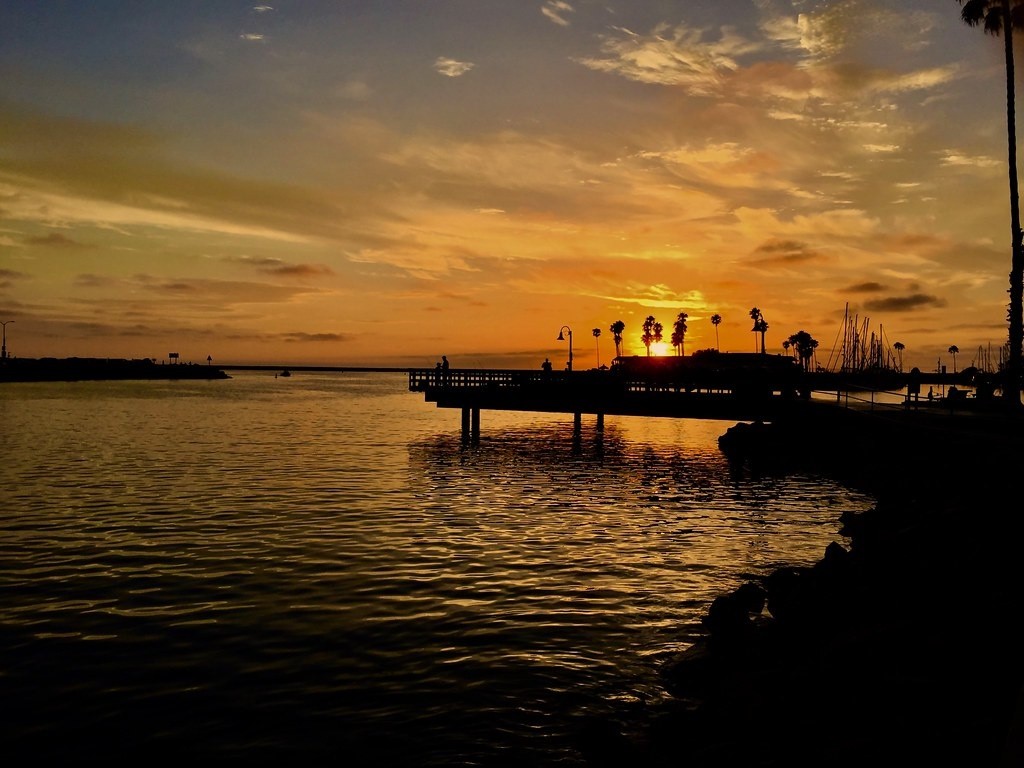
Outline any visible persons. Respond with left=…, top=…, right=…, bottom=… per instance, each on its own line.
left=435, top=355, right=450, bottom=387
left=541, top=357, right=552, bottom=369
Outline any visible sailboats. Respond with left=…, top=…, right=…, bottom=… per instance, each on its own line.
left=799, top=300, right=908, bottom=392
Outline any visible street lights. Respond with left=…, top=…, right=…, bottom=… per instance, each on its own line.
left=557, top=325, right=573, bottom=371
